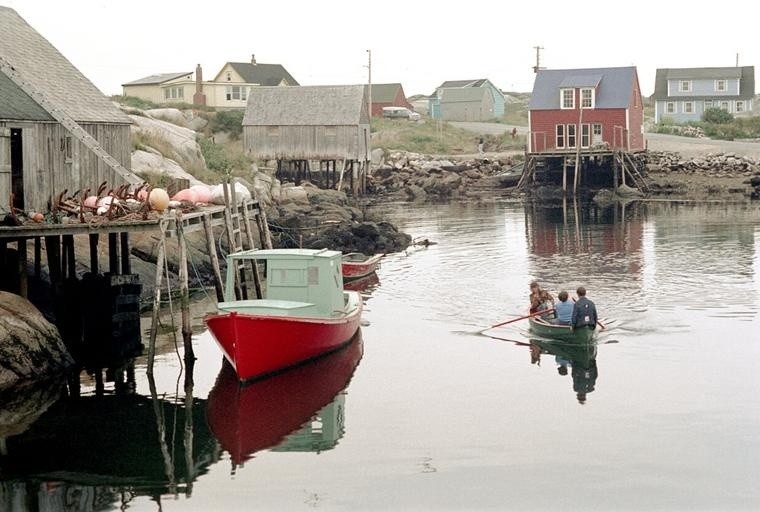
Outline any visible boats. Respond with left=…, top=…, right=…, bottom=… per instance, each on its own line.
left=530, top=309, right=596, bottom=345
left=204, top=250, right=364, bottom=385
left=341, top=253, right=385, bottom=276
left=207, top=329, right=368, bottom=475
left=472, top=296, right=605, bottom=345
left=198, top=245, right=366, bottom=384
left=342, top=253, right=387, bottom=280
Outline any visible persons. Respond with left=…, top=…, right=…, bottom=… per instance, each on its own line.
left=548, top=290, right=574, bottom=326
left=479, top=135, right=484, bottom=155
left=530, top=282, right=553, bottom=314
left=572, top=359, right=597, bottom=400
left=556, top=354, right=572, bottom=375
left=571, top=287, right=597, bottom=330
left=537, top=290, right=555, bottom=319
